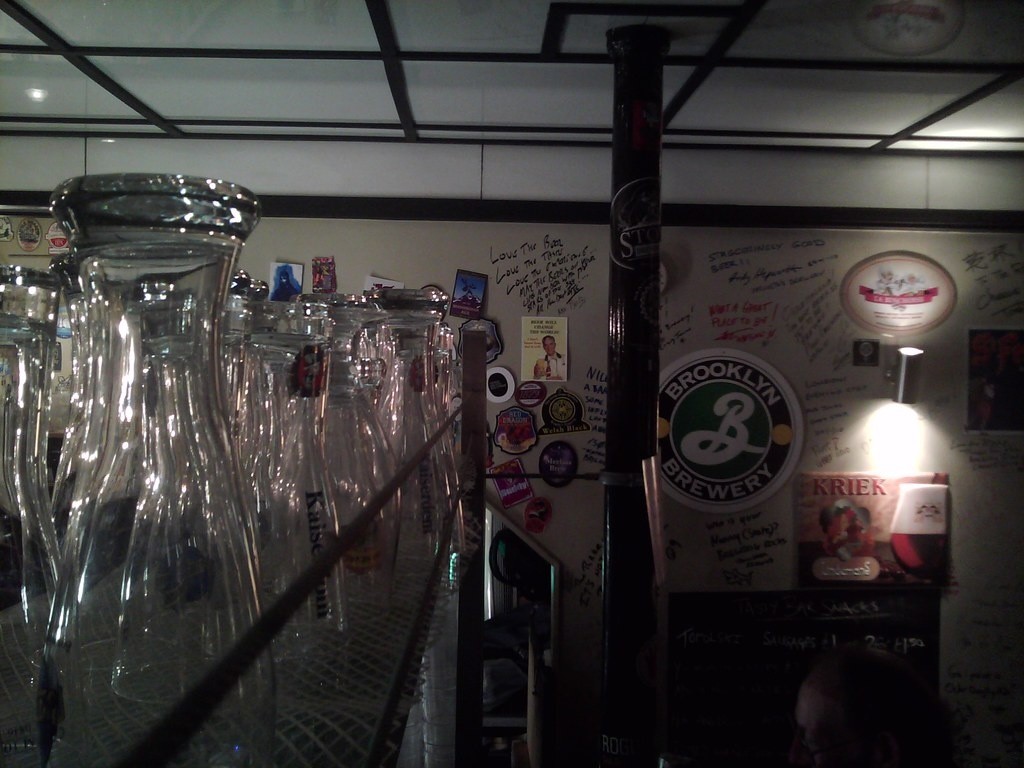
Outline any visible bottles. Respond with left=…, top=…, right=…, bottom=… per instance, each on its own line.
left=0, top=173, right=463, bottom=768
